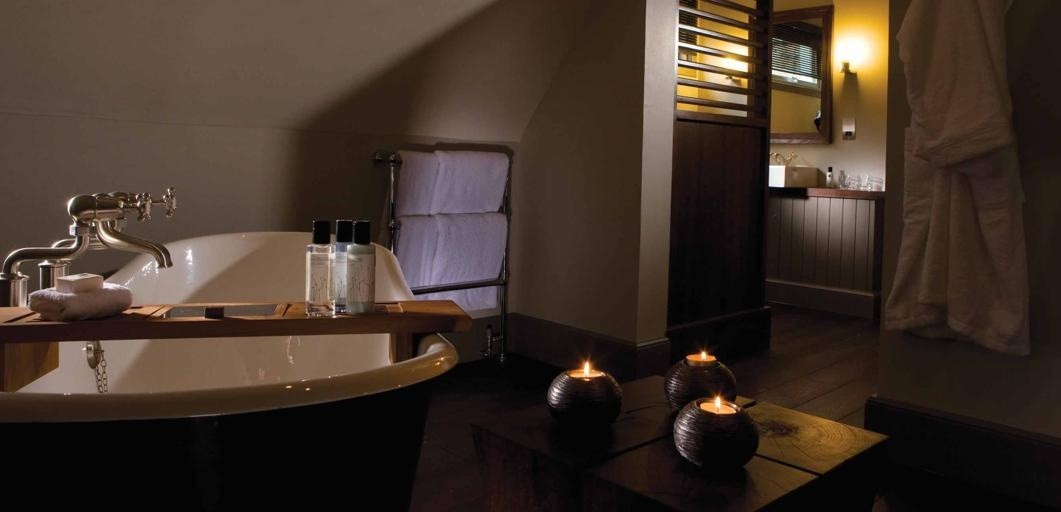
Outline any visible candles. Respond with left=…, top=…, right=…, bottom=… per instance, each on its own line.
left=545, top=353, right=759, bottom=472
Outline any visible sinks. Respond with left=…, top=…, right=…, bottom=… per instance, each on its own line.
left=768, top=164, right=818, bottom=188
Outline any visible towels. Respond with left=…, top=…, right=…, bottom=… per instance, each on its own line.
left=27, top=282, right=133, bottom=322
left=396, top=150, right=510, bottom=310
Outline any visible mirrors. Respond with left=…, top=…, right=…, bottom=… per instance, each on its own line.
left=746, top=3, right=834, bottom=146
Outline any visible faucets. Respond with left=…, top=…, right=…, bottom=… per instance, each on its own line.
left=769, top=152, right=797, bottom=164
left=3, top=190, right=176, bottom=271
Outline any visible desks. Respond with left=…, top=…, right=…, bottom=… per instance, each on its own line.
left=468, top=384, right=894, bottom=510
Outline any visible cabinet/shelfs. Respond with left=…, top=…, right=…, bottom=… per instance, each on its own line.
left=764, top=196, right=886, bottom=321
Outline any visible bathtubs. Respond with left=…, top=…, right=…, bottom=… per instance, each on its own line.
left=0, top=232, right=458, bottom=512
